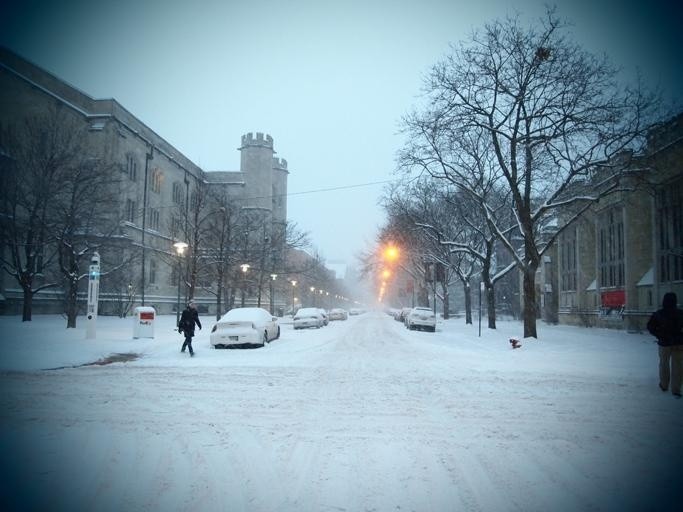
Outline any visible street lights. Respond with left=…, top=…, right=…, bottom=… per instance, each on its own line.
left=237, top=262, right=363, bottom=310
left=169, top=238, right=190, bottom=331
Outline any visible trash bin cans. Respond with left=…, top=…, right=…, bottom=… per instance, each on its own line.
left=133, top=307, right=156, bottom=339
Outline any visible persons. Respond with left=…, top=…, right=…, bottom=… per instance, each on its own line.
left=646, top=291, right=683, bottom=400
left=176, top=301, right=202, bottom=357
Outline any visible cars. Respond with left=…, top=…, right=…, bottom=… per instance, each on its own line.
left=292, top=307, right=366, bottom=331
left=208, top=307, right=282, bottom=349
left=382, top=306, right=437, bottom=332
